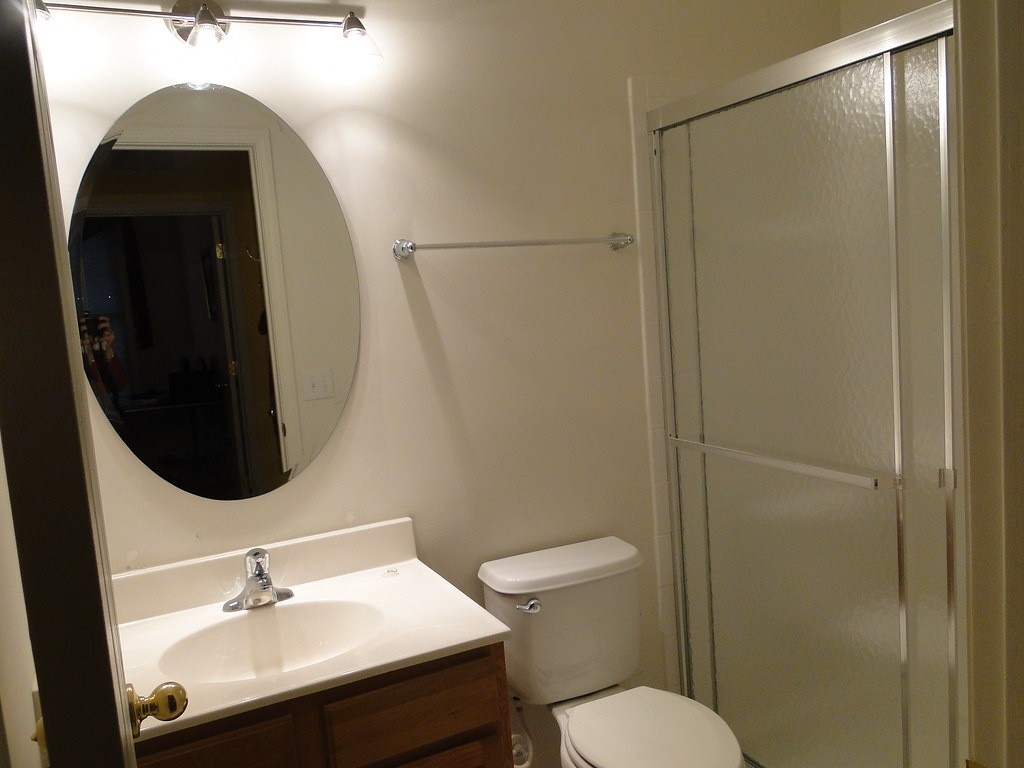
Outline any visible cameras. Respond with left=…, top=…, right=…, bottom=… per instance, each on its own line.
left=85, top=318, right=104, bottom=336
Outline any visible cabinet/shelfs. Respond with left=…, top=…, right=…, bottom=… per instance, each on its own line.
left=133, top=641, right=517, bottom=768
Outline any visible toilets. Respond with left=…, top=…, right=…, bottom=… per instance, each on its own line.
left=477, top=535, right=747, bottom=768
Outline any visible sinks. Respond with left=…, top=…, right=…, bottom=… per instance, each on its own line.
left=158, top=601, right=388, bottom=681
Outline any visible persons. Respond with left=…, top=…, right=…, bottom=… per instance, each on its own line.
left=80, top=315, right=127, bottom=397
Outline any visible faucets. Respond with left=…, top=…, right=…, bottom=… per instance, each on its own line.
left=222, top=548, right=293, bottom=612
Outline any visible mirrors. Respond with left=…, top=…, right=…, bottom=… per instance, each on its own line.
left=68, top=81, right=361, bottom=501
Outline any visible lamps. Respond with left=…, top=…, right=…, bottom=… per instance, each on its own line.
left=35, top=0, right=384, bottom=72
